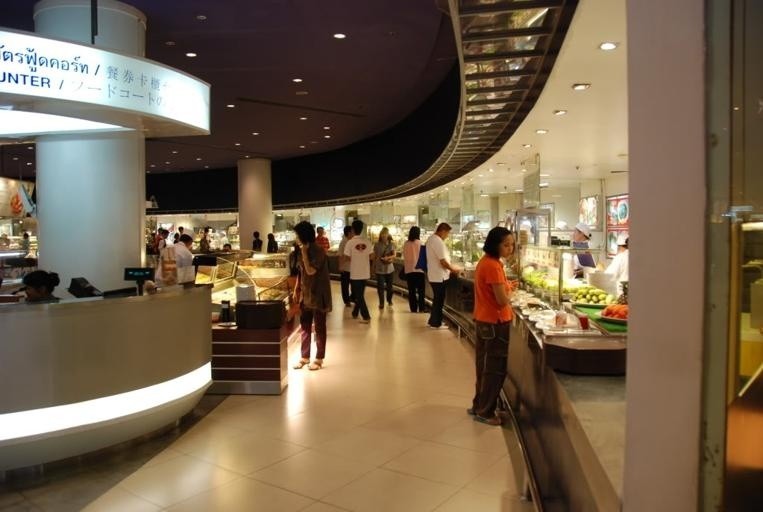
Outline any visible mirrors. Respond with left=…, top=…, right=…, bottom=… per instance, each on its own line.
left=457, top=7, right=554, bottom=150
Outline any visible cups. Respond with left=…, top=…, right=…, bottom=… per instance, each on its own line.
left=551, top=235, right=569, bottom=247
left=211, top=311, right=218, bottom=323
left=520, top=230, right=528, bottom=244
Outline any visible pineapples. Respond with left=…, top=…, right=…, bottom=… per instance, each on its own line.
left=618, top=294, right=628, bottom=304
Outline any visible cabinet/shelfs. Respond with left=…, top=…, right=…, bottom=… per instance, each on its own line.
left=190, top=250, right=304, bottom=397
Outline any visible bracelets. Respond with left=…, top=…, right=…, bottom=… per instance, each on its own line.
left=294, top=287, right=300, bottom=291
left=302, top=258, right=308, bottom=262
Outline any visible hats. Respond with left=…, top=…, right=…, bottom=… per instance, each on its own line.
left=616, top=234, right=628, bottom=246
left=182, top=228, right=196, bottom=240
left=576, top=222, right=592, bottom=239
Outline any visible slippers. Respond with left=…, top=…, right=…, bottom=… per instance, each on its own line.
left=466, top=406, right=504, bottom=425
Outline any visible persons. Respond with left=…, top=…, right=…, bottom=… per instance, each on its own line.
left=604, top=231, right=628, bottom=295
left=0, top=233, right=10, bottom=249
left=22, top=270, right=61, bottom=301
left=18, top=232, right=30, bottom=256
left=468, top=226, right=519, bottom=425
left=292, top=221, right=331, bottom=369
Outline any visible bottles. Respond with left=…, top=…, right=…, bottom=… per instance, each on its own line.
left=232, top=308, right=236, bottom=320
left=220, top=292, right=229, bottom=321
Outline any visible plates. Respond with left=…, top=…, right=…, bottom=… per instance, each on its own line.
left=617, top=200, right=628, bottom=224
left=508, top=280, right=626, bottom=335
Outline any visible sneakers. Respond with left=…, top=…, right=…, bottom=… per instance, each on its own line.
left=426, top=320, right=451, bottom=331
left=360, top=316, right=370, bottom=324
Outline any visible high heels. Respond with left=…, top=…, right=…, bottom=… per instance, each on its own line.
left=292, top=358, right=311, bottom=370
left=307, top=360, right=324, bottom=371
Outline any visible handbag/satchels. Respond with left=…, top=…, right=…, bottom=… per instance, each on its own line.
left=415, top=245, right=427, bottom=271
left=398, top=266, right=407, bottom=281
